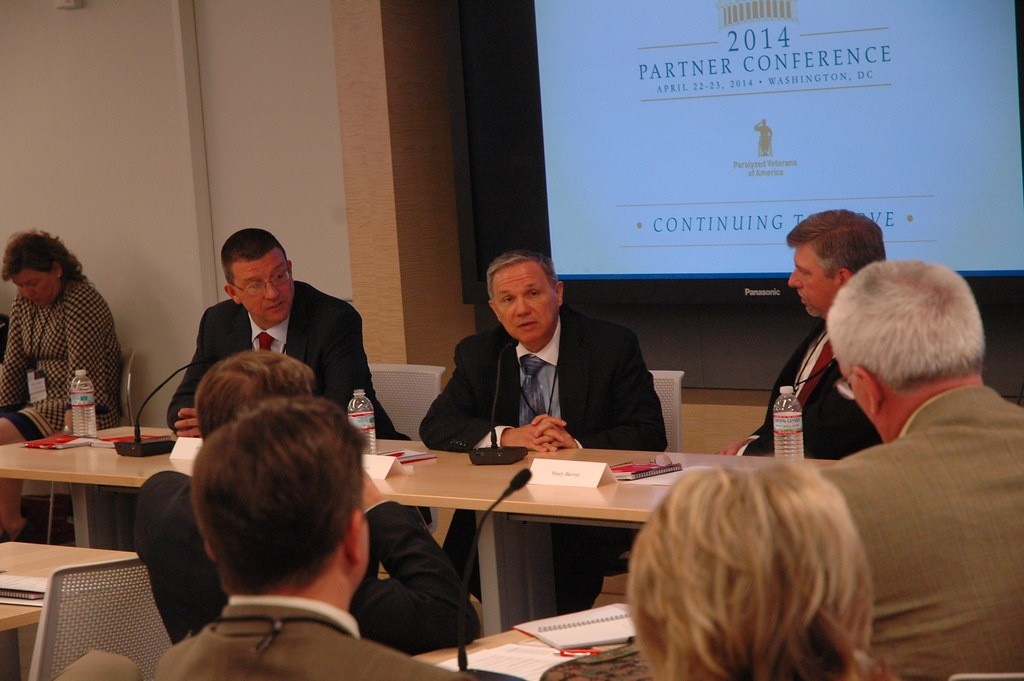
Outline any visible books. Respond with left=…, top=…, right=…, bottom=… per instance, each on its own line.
left=23, top=434, right=99, bottom=450
left=608, top=464, right=682, bottom=480
left=377, top=449, right=438, bottom=465
left=89, top=434, right=172, bottom=448
left=0, top=588, right=44, bottom=601
left=512, top=605, right=637, bottom=652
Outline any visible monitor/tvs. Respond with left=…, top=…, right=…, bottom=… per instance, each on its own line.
left=443, top=0, right=1024, bottom=309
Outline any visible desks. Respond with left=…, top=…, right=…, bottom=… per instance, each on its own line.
left=412, top=630, right=633, bottom=681
left=0, top=540, right=140, bottom=681
left=0, top=427, right=846, bottom=636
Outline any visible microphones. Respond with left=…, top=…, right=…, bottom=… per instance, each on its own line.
left=114, top=355, right=221, bottom=457
left=468, top=339, right=529, bottom=467
left=455, top=467, right=532, bottom=681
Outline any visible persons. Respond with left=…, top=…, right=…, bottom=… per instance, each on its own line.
left=717, top=208, right=887, bottom=459
left=152, top=390, right=478, bottom=681
left=51, top=649, right=145, bottom=681
left=826, top=261, right=1024, bottom=681
left=0, top=230, right=125, bottom=543
left=418, top=249, right=667, bottom=614
left=133, top=350, right=481, bottom=655
left=165, top=227, right=432, bottom=526
left=630, top=461, right=873, bottom=681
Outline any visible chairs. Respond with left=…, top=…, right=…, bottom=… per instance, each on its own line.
left=45, top=343, right=138, bottom=542
left=644, top=369, right=684, bottom=455
left=28, top=555, right=173, bottom=681
left=366, top=363, right=445, bottom=539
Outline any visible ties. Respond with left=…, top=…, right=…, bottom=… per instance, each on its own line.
left=795, top=340, right=834, bottom=404
left=255, top=331, right=275, bottom=352
left=517, top=353, right=548, bottom=428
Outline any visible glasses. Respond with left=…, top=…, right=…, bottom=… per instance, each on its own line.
left=230, top=264, right=292, bottom=295
left=830, top=366, right=860, bottom=399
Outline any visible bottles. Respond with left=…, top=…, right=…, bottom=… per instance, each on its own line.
left=69, top=370, right=98, bottom=438
left=347, top=390, right=377, bottom=455
left=772, top=386, right=804, bottom=462
